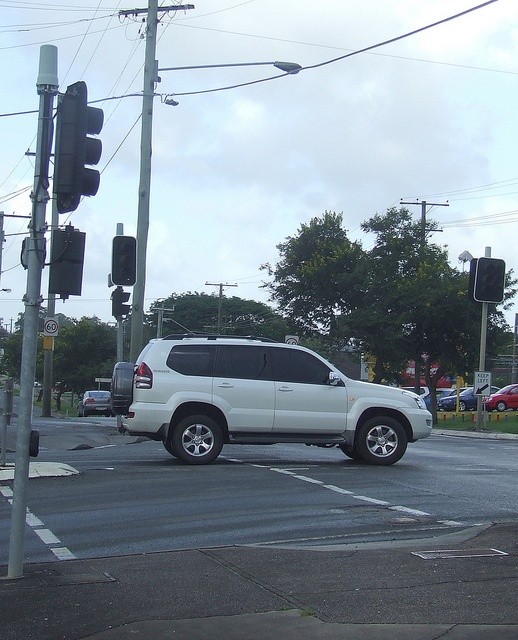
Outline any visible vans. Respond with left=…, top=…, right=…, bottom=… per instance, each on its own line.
left=399, top=387, right=430, bottom=399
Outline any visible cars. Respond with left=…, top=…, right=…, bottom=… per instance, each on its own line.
left=438, top=386, right=500, bottom=411
left=483, top=384, right=518, bottom=412
left=421, top=388, right=453, bottom=411
left=77, top=390, right=112, bottom=417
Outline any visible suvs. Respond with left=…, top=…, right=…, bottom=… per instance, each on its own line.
left=111, top=335, right=432, bottom=464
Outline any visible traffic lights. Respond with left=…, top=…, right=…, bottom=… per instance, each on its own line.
left=111, top=236, right=137, bottom=285
left=468, top=256, right=505, bottom=305
left=49, top=226, right=86, bottom=299
left=53, top=82, right=105, bottom=214
left=111, top=288, right=131, bottom=319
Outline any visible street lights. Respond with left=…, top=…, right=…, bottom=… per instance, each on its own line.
left=128, top=61, right=302, bottom=363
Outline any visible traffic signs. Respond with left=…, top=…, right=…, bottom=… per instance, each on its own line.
left=474, top=371, right=491, bottom=396
left=285, top=336, right=299, bottom=346
left=44, top=318, right=58, bottom=337
